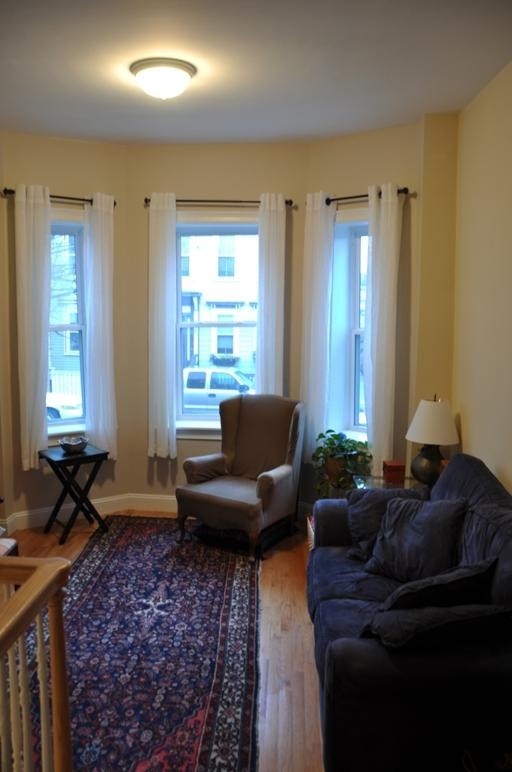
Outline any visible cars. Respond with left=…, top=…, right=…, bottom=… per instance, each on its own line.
left=46, top=391, right=83, bottom=421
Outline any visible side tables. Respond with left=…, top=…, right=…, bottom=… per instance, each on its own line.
left=353, top=474, right=404, bottom=489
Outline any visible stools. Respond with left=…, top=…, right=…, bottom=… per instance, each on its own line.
left=0, top=537, right=21, bottom=591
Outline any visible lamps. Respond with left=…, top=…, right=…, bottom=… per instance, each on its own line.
left=404, top=399, right=461, bottom=485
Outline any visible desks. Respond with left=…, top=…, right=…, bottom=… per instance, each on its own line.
left=39, top=443, right=109, bottom=545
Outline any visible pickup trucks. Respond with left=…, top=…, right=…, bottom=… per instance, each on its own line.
left=181, top=366, right=257, bottom=405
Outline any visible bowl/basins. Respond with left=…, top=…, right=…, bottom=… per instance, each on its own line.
left=57, top=435, right=89, bottom=452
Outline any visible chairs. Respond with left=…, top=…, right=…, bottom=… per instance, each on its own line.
left=175, top=394, right=308, bottom=561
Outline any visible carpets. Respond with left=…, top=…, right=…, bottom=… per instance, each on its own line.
left=0, top=515, right=261, bottom=772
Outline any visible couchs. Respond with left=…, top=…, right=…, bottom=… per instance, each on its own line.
left=307, top=454, right=511, bottom=771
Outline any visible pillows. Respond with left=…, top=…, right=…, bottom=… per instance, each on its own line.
left=372, top=602, right=508, bottom=653
left=360, top=554, right=500, bottom=643
left=365, top=499, right=469, bottom=581
left=345, top=485, right=433, bottom=560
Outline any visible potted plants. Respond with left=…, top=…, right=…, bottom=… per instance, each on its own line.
left=311, top=429, right=373, bottom=498
